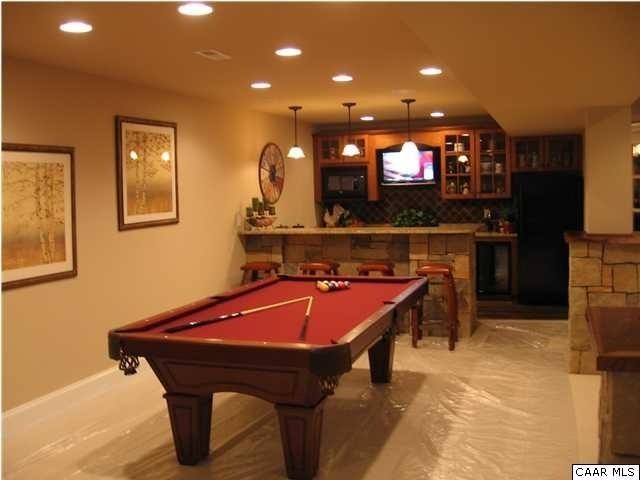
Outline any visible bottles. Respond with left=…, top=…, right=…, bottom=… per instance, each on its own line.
left=479, top=207, right=512, bottom=233
left=518, top=150, right=571, bottom=168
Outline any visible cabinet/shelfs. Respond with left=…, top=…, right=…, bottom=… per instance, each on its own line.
left=311, top=127, right=376, bottom=167
left=440, top=124, right=512, bottom=202
left=510, top=135, right=582, bottom=176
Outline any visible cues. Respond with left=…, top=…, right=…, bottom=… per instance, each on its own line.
left=166, top=295, right=314, bottom=343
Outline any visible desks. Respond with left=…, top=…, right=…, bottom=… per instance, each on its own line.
left=577, top=299, right=640, bottom=464
left=104, top=273, right=435, bottom=480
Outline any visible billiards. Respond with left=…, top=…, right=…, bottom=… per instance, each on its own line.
left=316, top=280, right=351, bottom=292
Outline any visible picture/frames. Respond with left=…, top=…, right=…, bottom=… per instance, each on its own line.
left=1, top=139, right=81, bottom=294
left=110, top=110, right=182, bottom=234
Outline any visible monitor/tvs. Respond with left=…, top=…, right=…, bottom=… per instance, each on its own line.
left=376, top=147, right=442, bottom=188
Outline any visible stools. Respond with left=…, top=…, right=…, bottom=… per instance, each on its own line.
left=353, top=259, right=397, bottom=280
left=292, top=259, right=345, bottom=277
left=408, top=262, right=462, bottom=352
left=239, top=260, right=283, bottom=288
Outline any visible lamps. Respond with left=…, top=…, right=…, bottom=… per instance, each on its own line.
left=338, top=100, right=362, bottom=159
left=399, top=95, right=423, bottom=159
left=286, top=102, right=307, bottom=162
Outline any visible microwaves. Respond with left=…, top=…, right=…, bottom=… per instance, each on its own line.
left=322, top=173, right=365, bottom=197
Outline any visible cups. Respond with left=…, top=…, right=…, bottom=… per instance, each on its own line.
left=445, top=135, right=504, bottom=195
